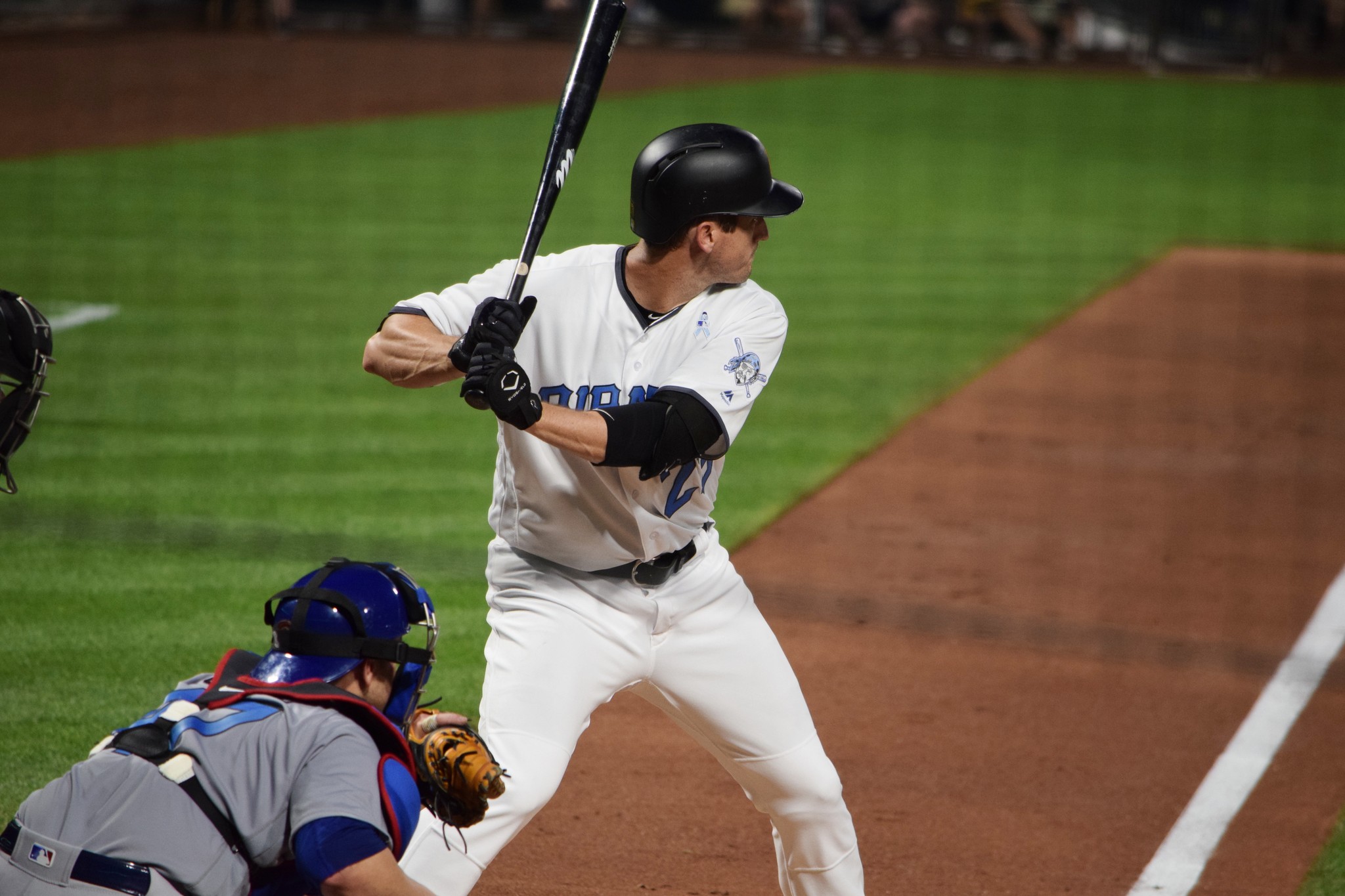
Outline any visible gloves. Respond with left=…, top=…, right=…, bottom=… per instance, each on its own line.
left=460, top=342, right=542, bottom=431
left=447, top=296, right=537, bottom=374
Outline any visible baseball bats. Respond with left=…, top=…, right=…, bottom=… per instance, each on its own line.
left=465, top=1, right=624, bottom=407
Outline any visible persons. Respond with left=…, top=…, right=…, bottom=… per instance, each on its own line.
left=0, top=290, right=52, bottom=494
left=0, top=557, right=507, bottom=896
left=361, top=124, right=865, bottom=896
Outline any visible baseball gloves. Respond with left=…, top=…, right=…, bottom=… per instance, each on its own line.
left=404, top=705, right=509, bottom=828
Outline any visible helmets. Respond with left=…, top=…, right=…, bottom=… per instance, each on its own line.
left=251, top=564, right=411, bottom=684
left=630, top=123, right=804, bottom=244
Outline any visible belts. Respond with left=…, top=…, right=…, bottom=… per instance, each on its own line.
left=0, top=820, right=151, bottom=896
left=586, top=523, right=707, bottom=585
